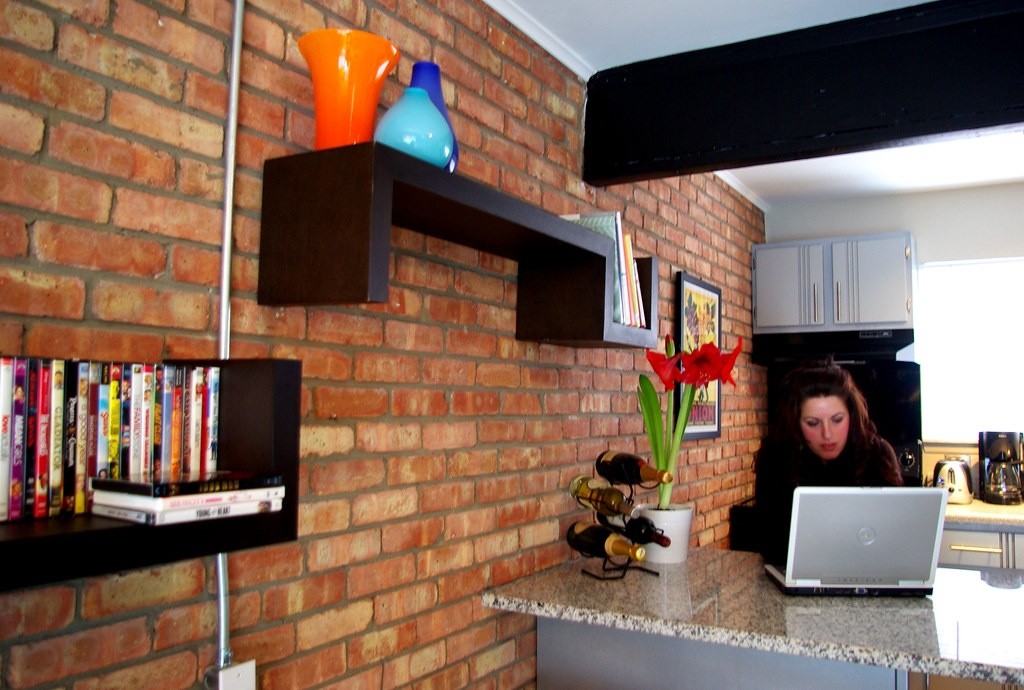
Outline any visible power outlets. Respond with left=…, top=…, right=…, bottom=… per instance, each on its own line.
left=944, top=453, right=971, bottom=466
left=203, top=659, right=257, bottom=690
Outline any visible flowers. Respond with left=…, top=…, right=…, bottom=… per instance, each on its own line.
left=637, top=333, right=744, bottom=508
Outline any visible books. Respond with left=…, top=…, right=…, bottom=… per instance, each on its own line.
left=0, top=355, right=286, bottom=526
left=560, top=212, right=646, bottom=328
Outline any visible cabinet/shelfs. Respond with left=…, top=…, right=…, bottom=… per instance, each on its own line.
left=933, top=493, right=1024, bottom=570
left=749, top=229, right=914, bottom=335
left=253, top=142, right=659, bottom=349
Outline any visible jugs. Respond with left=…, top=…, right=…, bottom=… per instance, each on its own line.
left=985, top=451, right=1022, bottom=496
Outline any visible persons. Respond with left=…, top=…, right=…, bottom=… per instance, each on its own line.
left=751, top=362, right=903, bottom=558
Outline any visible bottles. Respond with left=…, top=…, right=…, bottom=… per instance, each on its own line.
left=596, top=511, right=672, bottom=548
left=595, top=448, right=674, bottom=486
left=565, top=521, right=646, bottom=563
left=567, top=474, right=640, bottom=521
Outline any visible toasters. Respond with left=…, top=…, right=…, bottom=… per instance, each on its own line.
left=933, top=456, right=975, bottom=505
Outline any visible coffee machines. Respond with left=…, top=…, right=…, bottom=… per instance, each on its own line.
left=978, top=432, right=1024, bottom=504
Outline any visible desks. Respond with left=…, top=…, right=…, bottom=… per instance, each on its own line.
left=482, top=547, right=1024, bottom=690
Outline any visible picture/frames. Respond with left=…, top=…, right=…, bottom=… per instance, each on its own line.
left=674, top=270, right=723, bottom=442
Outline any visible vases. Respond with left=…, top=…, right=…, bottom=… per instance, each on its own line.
left=299, top=27, right=401, bottom=148
left=411, top=60, right=460, bottom=173
left=637, top=503, right=694, bottom=564
left=373, top=88, right=454, bottom=168
left=640, top=561, right=694, bottom=624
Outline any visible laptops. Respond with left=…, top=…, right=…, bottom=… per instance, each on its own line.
left=764, top=487, right=949, bottom=596
